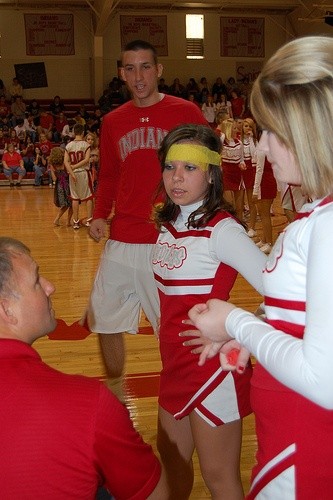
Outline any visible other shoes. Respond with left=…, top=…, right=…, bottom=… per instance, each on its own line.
left=247, top=229, right=257, bottom=238
left=72, top=218, right=80, bottom=229
left=9, top=182, right=21, bottom=187
left=256, top=240, right=273, bottom=255
left=85, top=216, right=94, bottom=227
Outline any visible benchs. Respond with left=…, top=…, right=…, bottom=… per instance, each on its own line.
left=0, top=98, right=123, bottom=186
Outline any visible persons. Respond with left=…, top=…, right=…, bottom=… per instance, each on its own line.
left=187, top=35, right=333, bottom=500
left=49, top=123, right=100, bottom=229
left=147, top=123, right=269, bottom=500
left=0, top=76, right=126, bottom=186
left=0, top=235, right=171, bottom=500
left=158, top=75, right=309, bottom=254
left=87, top=39, right=212, bottom=405
